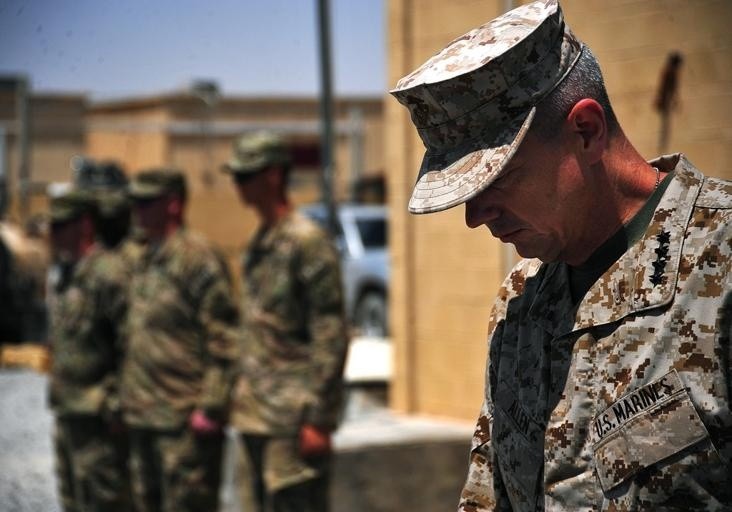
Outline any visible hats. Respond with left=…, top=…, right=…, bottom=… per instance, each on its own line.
left=389, top=0, right=584, bottom=214
left=220, top=132, right=296, bottom=174
left=47, top=162, right=185, bottom=223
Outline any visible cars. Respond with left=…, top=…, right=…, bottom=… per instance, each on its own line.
left=299, top=202, right=391, bottom=338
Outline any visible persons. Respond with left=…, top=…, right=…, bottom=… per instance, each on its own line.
left=113, top=168, right=244, bottom=512
left=391, top=0, right=731, bottom=511
left=218, top=128, right=353, bottom=512
left=34, top=182, right=135, bottom=512
